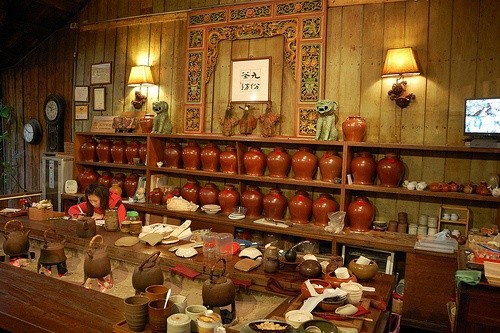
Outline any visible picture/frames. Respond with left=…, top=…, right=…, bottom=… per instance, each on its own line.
left=91, top=116, right=117, bottom=133
left=93, top=87, right=107, bottom=112
left=73, top=85, right=90, bottom=103
left=90, top=61, right=112, bottom=86
left=229, top=56, right=273, bottom=105
left=341, top=244, right=395, bottom=275
left=74, top=104, right=90, bottom=120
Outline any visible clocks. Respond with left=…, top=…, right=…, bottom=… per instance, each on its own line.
left=43, top=94, right=66, bottom=157
left=21, top=119, right=43, bottom=145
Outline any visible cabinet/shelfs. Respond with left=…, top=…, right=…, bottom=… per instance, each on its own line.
left=452, top=250, right=500, bottom=333
left=59, top=130, right=500, bottom=257
left=438, top=204, right=471, bottom=239
left=42, top=155, right=74, bottom=212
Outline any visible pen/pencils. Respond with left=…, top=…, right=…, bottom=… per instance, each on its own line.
left=78, top=206, right=83, bottom=213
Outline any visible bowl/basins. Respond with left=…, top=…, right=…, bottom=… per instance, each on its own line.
left=239, top=256, right=378, bottom=333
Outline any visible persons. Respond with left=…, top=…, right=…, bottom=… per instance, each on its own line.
left=68, top=184, right=126, bottom=222
left=220, top=101, right=278, bottom=137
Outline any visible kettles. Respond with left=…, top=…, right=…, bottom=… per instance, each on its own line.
left=3, top=220, right=31, bottom=256
left=202, top=258, right=236, bottom=308
left=76, top=213, right=96, bottom=238
left=84, top=234, right=111, bottom=278
left=132, top=251, right=164, bottom=292
left=38, top=228, right=67, bottom=265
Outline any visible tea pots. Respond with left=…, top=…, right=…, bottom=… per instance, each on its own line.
left=403, top=179, right=500, bottom=197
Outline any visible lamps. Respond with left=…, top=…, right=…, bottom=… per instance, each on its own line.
left=127, top=64, right=154, bottom=110
left=381, top=47, right=421, bottom=109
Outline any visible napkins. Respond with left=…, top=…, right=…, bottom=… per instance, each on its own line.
left=323, top=286, right=348, bottom=298
left=140, top=225, right=166, bottom=247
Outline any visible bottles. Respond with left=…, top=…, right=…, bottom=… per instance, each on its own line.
left=263, top=246, right=279, bottom=273
left=139, top=114, right=154, bottom=133
left=78, top=138, right=342, bottom=254
left=342, top=116, right=366, bottom=142
left=442, top=213, right=460, bottom=238
left=351, top=151, right=405, bottom=188
left=285, top=248, right=296, bottom=262
left=346, top=195, right=374, bottom=232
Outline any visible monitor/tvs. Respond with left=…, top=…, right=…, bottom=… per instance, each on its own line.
left=463, top=97, right=500, bottom=137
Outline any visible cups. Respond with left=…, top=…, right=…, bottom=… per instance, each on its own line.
left=120, top=221, right=130, bottom=233
left=130, top=221, right=142, bottom=237
left=372, top=213, right=438, bottom=237
left=123, top=285, right=226, bottom=333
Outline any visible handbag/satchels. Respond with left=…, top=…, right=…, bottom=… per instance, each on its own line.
left=148, top=171, right=168, bottom=202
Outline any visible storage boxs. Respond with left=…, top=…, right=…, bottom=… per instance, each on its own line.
left=203, top=232, right=234, bottom=265
left=28, top=207, right=54, bottom=222
left=392, top=298, right=404, bottom=316
